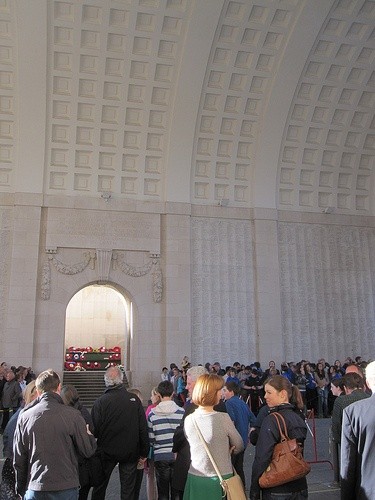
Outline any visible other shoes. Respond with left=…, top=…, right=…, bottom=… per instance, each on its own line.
left=327, top=480, right=339, bottom=489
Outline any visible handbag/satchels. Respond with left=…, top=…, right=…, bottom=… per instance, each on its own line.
left=221, top=474, right=248, bottom=500
left=258, top=412, right=311, bottom=487
